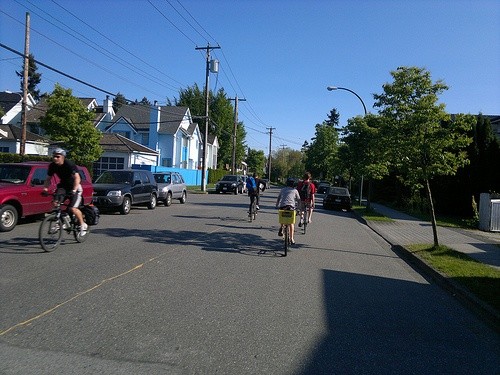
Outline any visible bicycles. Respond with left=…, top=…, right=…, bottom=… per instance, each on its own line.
left=247, top=189, right=263, bottom=221
left=298, top=204, right=310, bottom=234
left=38, top=191, right=90, bottom=252
left=273, top=206, right=300, bottom=256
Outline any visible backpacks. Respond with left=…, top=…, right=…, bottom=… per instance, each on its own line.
left=301, top=185, right=311, bottom=199
left=246, top=177, right=257, bottom=191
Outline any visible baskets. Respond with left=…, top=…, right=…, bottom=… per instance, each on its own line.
left=278, top=209, right=295, bottom=224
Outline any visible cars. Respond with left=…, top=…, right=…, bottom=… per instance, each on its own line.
left=323, top=186, right=352, bottom=209
left=317, top=183, right=330, bottom=193
left=259, top=179, right=270, bottom=190
left=152, top=172, right=188, bottom=207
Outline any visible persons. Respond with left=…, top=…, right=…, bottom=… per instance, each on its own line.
left=248, top=173, right=266, bottom=217
left=297, top=172, right=315, bottom=227
left=276, top=177, right=301, bottom=244
left=43, top=148, right=88, bottom=236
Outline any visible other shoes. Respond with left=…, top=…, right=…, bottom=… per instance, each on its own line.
left=278, top=227, right=283, bottom=236
left=256, top=207, right=260, bottom=210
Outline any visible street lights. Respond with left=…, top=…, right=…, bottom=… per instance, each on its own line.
left=327, top=85, right=368, bottom=206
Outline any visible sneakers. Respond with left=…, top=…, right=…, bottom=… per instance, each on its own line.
left=50, top=223, right=66, bottom=229
left=79, top=222, right=88, bottom=237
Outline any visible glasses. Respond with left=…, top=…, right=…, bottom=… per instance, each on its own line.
left=53, top=155, right=60, bottom=158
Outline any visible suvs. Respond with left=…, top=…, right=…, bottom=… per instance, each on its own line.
left=241, top=175, right=248, bottom=193
left=216, top=174, right=244, bottom=195
left=92, top=168, right=158, bottom=215
left=0, top=160, right=94, bottom=232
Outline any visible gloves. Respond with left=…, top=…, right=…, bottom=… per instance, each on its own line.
left=40, top=191, right=48, bottom=196
left=69, top=190, right=76, bottom=196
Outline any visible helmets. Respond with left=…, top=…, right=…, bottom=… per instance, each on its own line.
left=53, top=148, right=66, bottom=156
